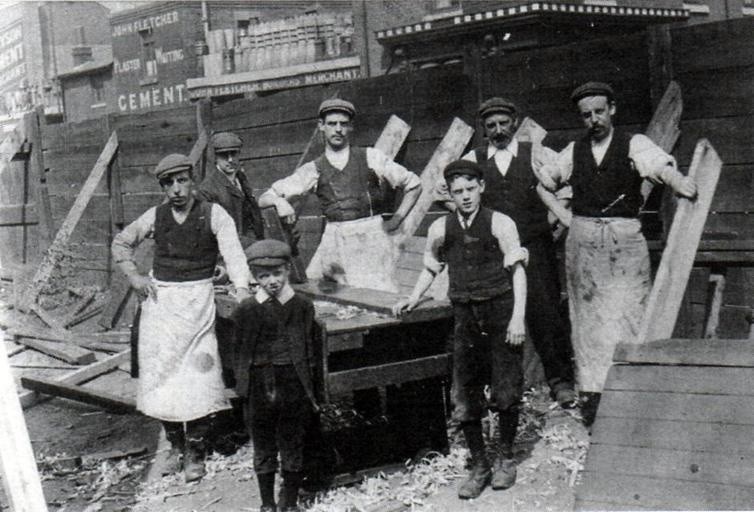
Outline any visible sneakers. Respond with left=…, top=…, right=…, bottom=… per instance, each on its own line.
left=444, top=161, right=483, bottom=177
left=478, top=97, right=517, bottom=115
left=317, top=99, right=355, bottom=116
left=577, top=392, right=601, bottom=425
left=571, top=82, right=614, bottom=101
left=246, top=239, right=291, bottom=266
left=555, top=388, right=575, bottom=406
left=212, top=132, right=242, bottom=153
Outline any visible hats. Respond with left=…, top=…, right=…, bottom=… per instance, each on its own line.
left=156, top=154, right=191, bottom=178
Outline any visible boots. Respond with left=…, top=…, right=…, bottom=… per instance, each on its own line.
left=258, top=472, right=277, bottom=511
left=185, top=427, right=206, bottom=482
left=162, top=430, right=187, bottom=475
left=279, top=470, right=300, bottom=511
left=490, top=412, right=518, bottom=490
left=458, top=421, right=491, bottom=499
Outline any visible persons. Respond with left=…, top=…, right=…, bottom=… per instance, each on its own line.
left=390, top=158, right=530, bottom=500
left=535, top=80, right=698, bottom=435
left=430, top=93, right=574, bottom=407
left=108, top=152, right=255, bottom=482
left=192, top=131, right=264, bottom=280
left=229, top=239, right=318, bottom=511
left=258, top=96, right=421, bottom=290
